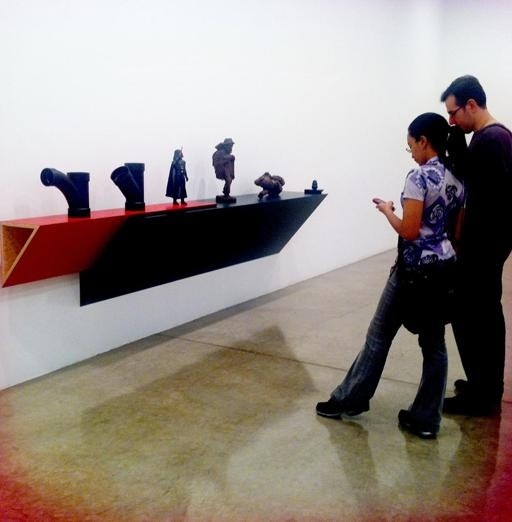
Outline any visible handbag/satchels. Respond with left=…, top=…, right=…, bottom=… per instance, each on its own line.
left=390, top=263, right=453, bottom=335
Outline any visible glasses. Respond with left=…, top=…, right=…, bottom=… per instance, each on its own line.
left=447, top=105, right=467, bottom=117
left=405, top=137, right=417, bottom=154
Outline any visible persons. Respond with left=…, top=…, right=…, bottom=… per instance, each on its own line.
left=214, top=136, right=236, bottom=198
left=166, top=147, right=189, bottom=206
left=438, top=72, right=511, bottom=415
left=316, top=110, right=466, bottom=440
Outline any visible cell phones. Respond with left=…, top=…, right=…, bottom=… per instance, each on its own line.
left=372, top=197, right=395, bottom=211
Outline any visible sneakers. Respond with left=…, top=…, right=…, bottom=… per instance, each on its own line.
left=442, top=379, right=503, bottom=417
left=399, top=406, right=442, bottom=436
left=315, top=394, right=371, bottom=419
